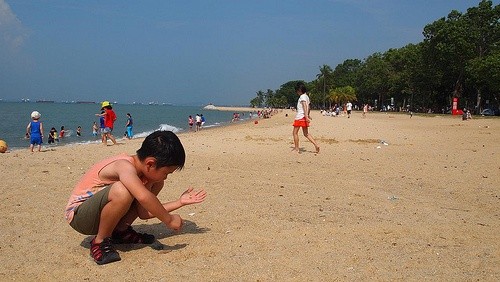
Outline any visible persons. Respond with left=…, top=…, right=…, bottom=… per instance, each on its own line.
left=76, top=126, right=81, bottom=137
left=128, top=114, right=133, bottom=139
left=65, top=130, right=208, bottom=264
left=27, top=110, right=44, bottom=154
left=318, top=95, right=500, bottom=122
left=92, top=121, right=100, bottom=137
left=58, top=125, right=69, bottom=141
left=125, top=113, right=134, bottom=140
left=99, top=111, right=110, bottom=146
left=25, top=132, right=31, bottom=139
left=47, top=127, right=55, bottom=145
left=186, top=112, right=207, bottom=131
left=230, top=102, right=298, bottom=123
left=291, top=81, right=320, bottom=154
left=123, top=130, right=129, bottom=139
left=49, top=128, right=59, bottom=146
left=94, top=100, right=118, bottom=146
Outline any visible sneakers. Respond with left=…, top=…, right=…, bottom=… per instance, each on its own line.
left=88, top=234, right=120, bottom=265
left=112, top=228, right=157, bottom=248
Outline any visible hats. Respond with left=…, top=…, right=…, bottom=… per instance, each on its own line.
left=100, top=101, right=112, bottom=110
left=31, top=111, right=43, bottom=120
